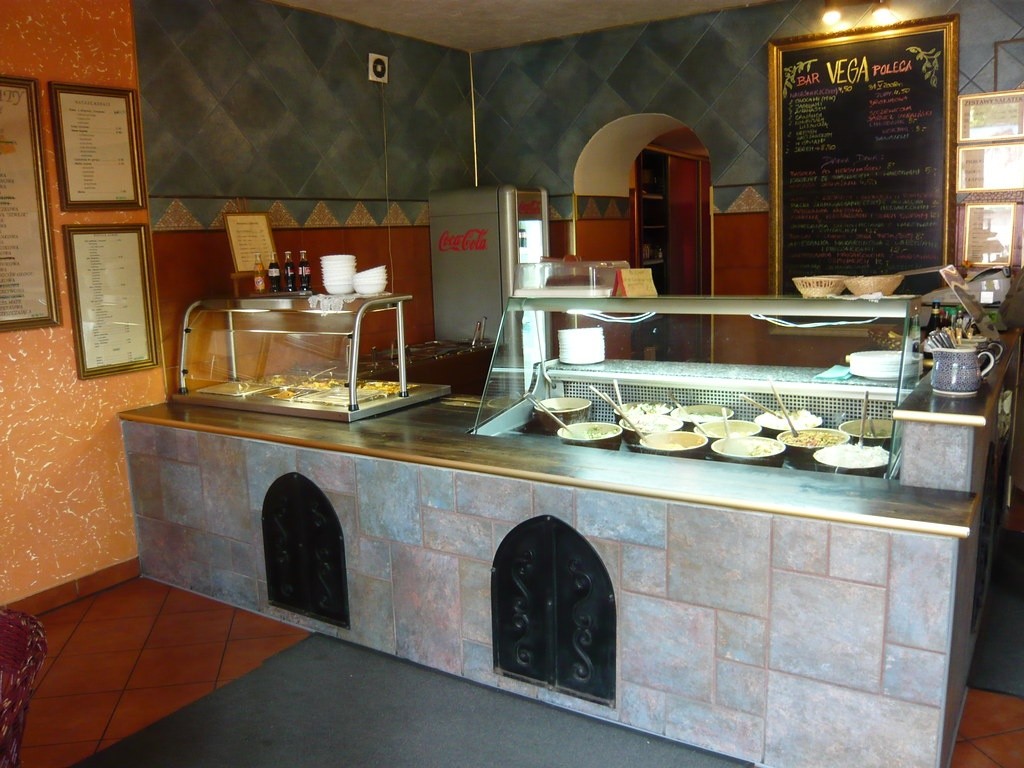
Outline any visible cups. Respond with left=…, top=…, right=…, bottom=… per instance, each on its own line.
left=929, top=335, right=1002, bottom=394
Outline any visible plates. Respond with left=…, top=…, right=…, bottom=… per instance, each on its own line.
left=849, top=351, right=923, bottom=382
left=557, top=328, right=605, bottom=365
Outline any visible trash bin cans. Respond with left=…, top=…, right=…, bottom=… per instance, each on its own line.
left=0, top=605, right=46, bottom=768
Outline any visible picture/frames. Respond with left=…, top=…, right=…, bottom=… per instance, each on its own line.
left=955, top=142, right=1024, bottom=193
left=963, top=201, right=1015, bottom=267
left=46, top=81, right=147, bottom=208
left=62, top=222, right=160, bottom=380
left=956, top=88, right=1024, bottom=143
left=0, top=73, right=62, bottom=333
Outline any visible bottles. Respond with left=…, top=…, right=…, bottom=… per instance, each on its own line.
left=254, top=252, right=266, bottom=295
left=923, top=300, right=964, bottom=359
left=298, top=250, right=311, bottom=290
left=283, top=251, right=296, bottom=291
left=268, top=252, right=282, bottom=293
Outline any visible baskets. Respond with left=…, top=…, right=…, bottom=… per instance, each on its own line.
left=843, top=274, right=904, bottom=296
left=792, top=274, right=850, bottom=298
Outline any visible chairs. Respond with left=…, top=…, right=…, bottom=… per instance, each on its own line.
left=0, top=608, right=48, bottom=768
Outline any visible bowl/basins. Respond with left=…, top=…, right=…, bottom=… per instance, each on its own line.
left=320, top=255, right=388, bottom=294
left=533, top=396, right=894, bottom=478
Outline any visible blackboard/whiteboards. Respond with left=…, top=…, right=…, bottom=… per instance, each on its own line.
left=769, top=12, right=960, bottom=296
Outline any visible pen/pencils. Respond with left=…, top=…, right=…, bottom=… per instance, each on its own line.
left=927, top=327, right=957, bottom=349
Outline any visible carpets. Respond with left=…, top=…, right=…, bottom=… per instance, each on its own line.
left=60, top=633, right=754, bottom=768
left=967, top=527, right=1023, bottom=699
